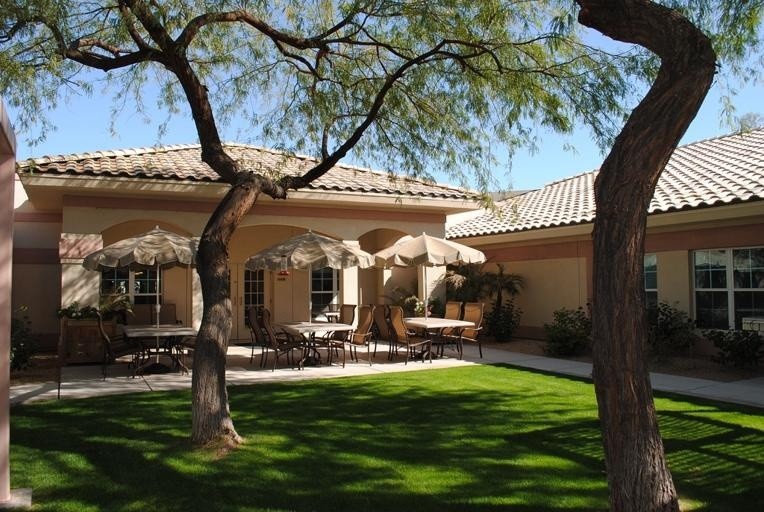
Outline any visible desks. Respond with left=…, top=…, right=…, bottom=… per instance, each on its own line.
left=275, top=321, right=354, bottom=370
left=119, top=319, right=196, bottom=378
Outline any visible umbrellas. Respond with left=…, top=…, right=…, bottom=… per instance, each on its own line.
left=83, top=223, right=198, bottom=362
left=241, top=228, right=374, bottom=354
left=370, top=230, right=487, bottom=348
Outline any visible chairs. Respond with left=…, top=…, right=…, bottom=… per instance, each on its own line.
left=319, top=300, right=486, bottom=369
left=92, top=307, right=144, bottom=381
left=247, top=305, right=301, bottom=370
